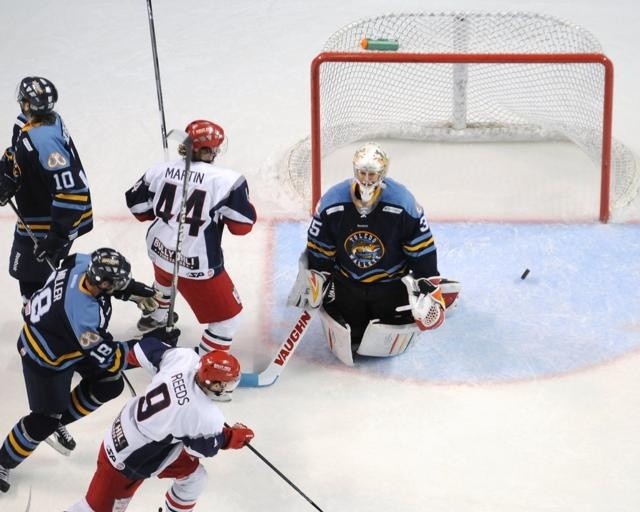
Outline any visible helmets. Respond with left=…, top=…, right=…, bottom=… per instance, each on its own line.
left=354, top=142, right=389, bottom=205
left=87, top=249, right=130, bottom=288
left=196, top=350, right=242, bottom=395
left=17, top=77, right=57, bottom=116
left=186, top=120, right=227, bottom=157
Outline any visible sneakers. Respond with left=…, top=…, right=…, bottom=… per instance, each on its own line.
left=55, top=423, right=76, bottom=450
left=0, top=464, right=10, bottom=493
left=138, top=312, right=179, bottom=331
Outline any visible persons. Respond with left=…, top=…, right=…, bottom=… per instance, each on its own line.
left=125, top=118, right=257, bottom=360
left=0, top=76, right=95, bottom=307
left=0, top=245, right=163, bottom=493
left=286, top=141, right=460, bottom=365
left=62, top=336, right=255, bottom=512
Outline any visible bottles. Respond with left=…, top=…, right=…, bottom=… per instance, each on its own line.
left=360, top=38, right=399, bottom=51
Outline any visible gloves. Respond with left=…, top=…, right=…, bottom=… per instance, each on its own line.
left=222, top=422, right=253, bottom=448
left=34, top=232, right=73, bottom=263
left=113, top=279, right=163, bottom=310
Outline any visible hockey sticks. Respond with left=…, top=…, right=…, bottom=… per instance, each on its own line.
left=166, top=126, right=194, bottom=333
left=238, top=305, right=318, bottom=388
